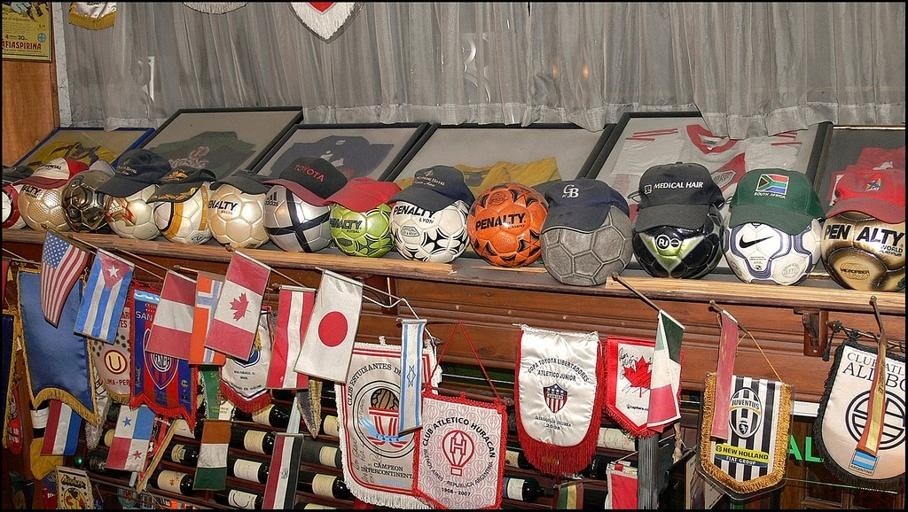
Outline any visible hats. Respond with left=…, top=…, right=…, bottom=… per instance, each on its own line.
left=826, top=163, right=906, bottom=224
left=260, top=155, right=348, bottom=207
left=540, top=178, right=630, bottom=235
left=389, top=164, right=476, bottom=212
left=12, top=156, right=89, bottom=191
left=145, top=165, right=217, bottom=205
left=634, top=160, right=725, bottom=233
left=94, top=148, right=172, bottom=199
left=321, top=176, right=402, bottom=213
left=728, top=167, right=826, bottom=236
left=209, top=169, right=275, bottom=195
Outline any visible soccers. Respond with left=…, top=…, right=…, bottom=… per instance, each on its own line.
left=389, top=200, right=470, bottom=264
left=103, top=183, right=160, bottom=240
left=540, top=204, right=632, bottom=286
left=721, top=211, right=822, bottom=287
left=2, top=182, right=26, bottom=229
left=330, top=203, right=394, bottom=257
left=62, top=178, right=112, bottom=234
left=17, top=182, right=71, bottom=233
left=466, top=182, right=549, bottom=269
left=153, top=182, right=212, bottom=246
left=207, top=185, right=270, bottom=249
left=263, top=184, right=333, bottom=252
left=822, top=210, right=908, bottom=294
left=632, top=203, right=724, bottom=278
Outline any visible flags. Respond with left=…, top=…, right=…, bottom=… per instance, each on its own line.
left=692, top=366, right=792, bottom=506
left=54, top=462, right=96, bottom=509
left=603, top=460, right=639, bottom=509
left=602, top=331, right=680, bottom=443
left=294, top=268, right=365, bottom=386
left=104, top=403, right=156, bottom=473
left=645, top=310, right=686, bottom=427
left=334, top=338, right=439, bottom=509
left=410, top=392, right=509, bottom=510
left=29, top=468, right=59, bottom=509
left=261, top=433, right=305, bottom=509
left=507, top=322, right=606, bottom=478
left=857, top=326, right=888, bottom=457
left=709, top=311, right=738, bottom=441
left=39, top=399, right=82, bottom=458
left=811, top=336, right=906, bottom=489
left=555, top=479, right=584, bottom=510
left=193, top=419, right=233, bottom=492
left=396, top=319, right=427, bottom=436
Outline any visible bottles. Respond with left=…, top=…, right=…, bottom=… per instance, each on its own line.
left=301, top=412, right=341, bottom=437
left=85, top=403, right=159, bottom=482
left=502, top=473, right=558, bottom=503
left=579, top=457, right=637, bottom=480
left=301, top=440, right=342, bottom=469
left=597, top=426, right=637, bottom=452
left=211, top=390, right=297, bottom=509
left=297, top=472, right=355, bottom=499
left=504, top=441, right=534, bottom=469
left=150, top=403, right=206, bottom=497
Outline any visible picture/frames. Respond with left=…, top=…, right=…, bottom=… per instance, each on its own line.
left=385, top=124, right=615, bottom=183
left=251, top=125, right=428, bottom=185
left=138, top=108, right=305, bottom=174
left=587, top=112, right=828, bottom=193
left=812, top=121, right=907, bottom=222
left=12, top=123, right=154, bottom=179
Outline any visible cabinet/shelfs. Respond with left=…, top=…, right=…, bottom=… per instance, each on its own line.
left=64, top=377, right=732, bottom=511
left=0, top=230, right=908, bottom=512
